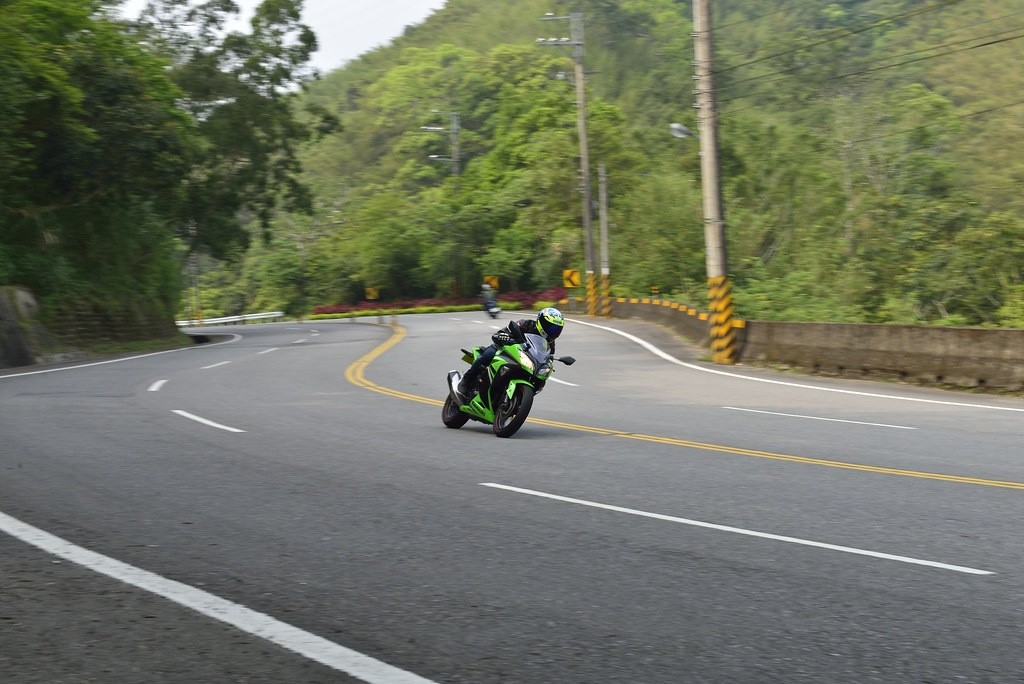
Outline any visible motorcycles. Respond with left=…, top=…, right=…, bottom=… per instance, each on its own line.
left=442, top=320, right=576, bottom=438
left=481, top=295, right=503, bottom=320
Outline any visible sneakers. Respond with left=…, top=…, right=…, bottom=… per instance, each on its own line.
left=456, top=375, right=475, bottom=398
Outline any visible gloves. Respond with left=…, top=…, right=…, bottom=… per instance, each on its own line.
left=498, top=332, right=510, bottom=345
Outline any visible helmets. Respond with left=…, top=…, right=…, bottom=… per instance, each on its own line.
left=535, top=307, right=565, bottom=345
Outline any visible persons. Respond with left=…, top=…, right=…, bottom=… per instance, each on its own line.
left=477, top=283, right=498, bottom=309
left=458, top=307, right=564, bottom=402
